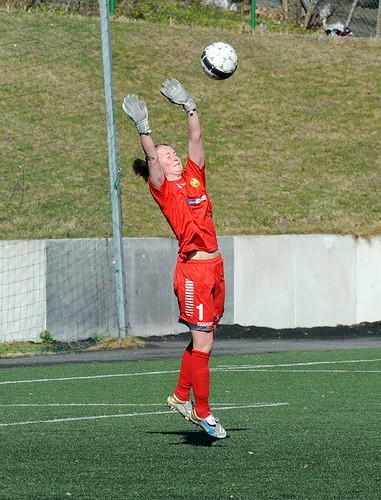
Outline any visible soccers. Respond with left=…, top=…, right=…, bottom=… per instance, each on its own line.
left=199, top=41, right=239, bottom=81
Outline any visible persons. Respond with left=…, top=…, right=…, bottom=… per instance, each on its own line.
left=122, top=77, right=226, bottom=438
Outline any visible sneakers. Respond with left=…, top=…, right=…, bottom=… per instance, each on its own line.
left=166, top=393, right=194, bottom=421
left=190, top=408, right=226, bottom=437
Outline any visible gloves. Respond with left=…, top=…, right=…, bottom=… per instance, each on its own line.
left=122, top=93, right=152, bottom=135
left=159, top=78, right=197, bottom=114
